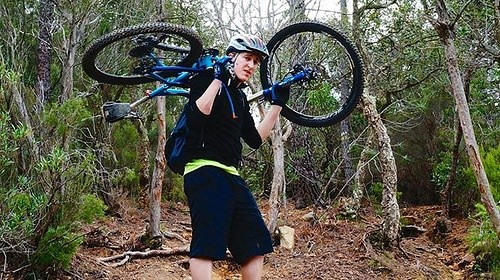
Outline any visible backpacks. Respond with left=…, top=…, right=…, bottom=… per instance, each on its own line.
left=165, top=104, right=194, bottom=175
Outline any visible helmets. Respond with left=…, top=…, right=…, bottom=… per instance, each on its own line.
left=226, top=33, right=270, bottom=63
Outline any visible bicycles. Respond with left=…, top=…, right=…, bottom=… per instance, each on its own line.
left=82, top=21, right=364, bottom=128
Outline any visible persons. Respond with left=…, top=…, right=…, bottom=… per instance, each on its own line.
left=183, top=34, right=290, bottom=280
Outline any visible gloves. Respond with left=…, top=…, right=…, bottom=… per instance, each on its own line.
left=273, top=80, right=290, bottom=108
left=213, top=56, right=235, bottom=84
